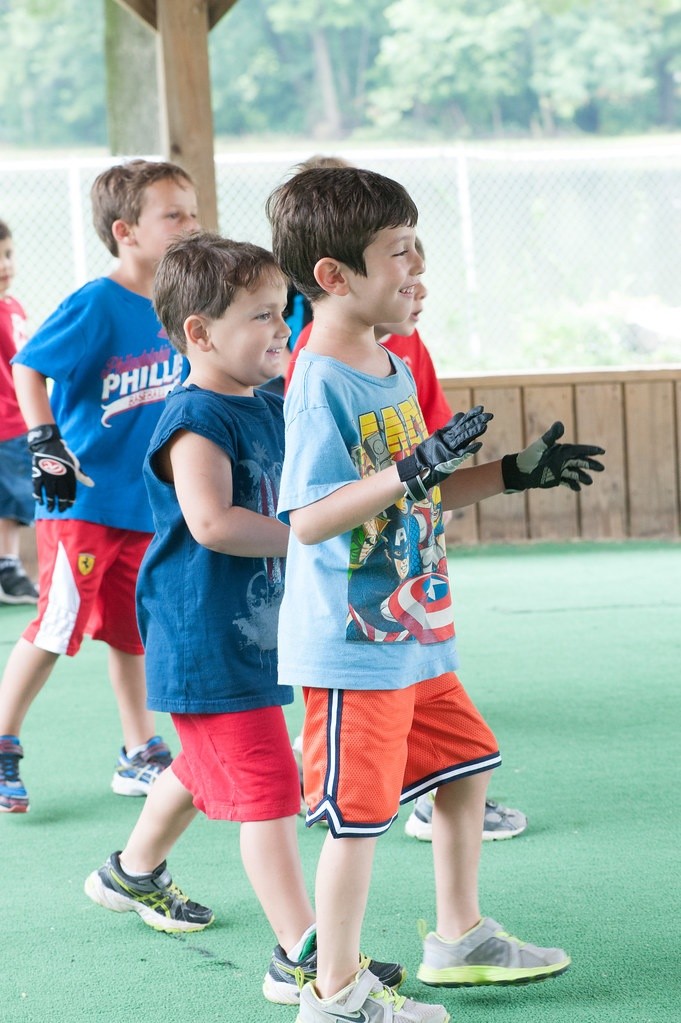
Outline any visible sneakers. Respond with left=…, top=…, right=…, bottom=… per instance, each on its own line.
left=417, top=915, right=570, bottom=988
left=262, top=927, right=406, bottom=1006
left=111, top=736, right=176, bottom=796
left=0, top=734, right=31, bottom=813
left=404, top=789, right=528, bottom=842
left=291, top=749, right=329, bottom=827
left=0, top=559, right=40, bottom=605
left=85, top=850, right=215, bottom=932
left=294, top=967, right=451, bottom=1023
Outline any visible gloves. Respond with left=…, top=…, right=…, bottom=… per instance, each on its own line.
left=27, top=423, right=95, bottom=512
left=396, top=404, right=494, bottom=500
left=501, top=420, right=605, bottom=495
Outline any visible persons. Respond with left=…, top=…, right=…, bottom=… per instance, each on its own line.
left=275, top=155, right=528, bottom=843
left=0, top=222, right=45, bottom=604
left=0, top=160, right=199, bottom=814
left=266, top=167, right=604, bottom=1023
left=83, top=231, right=402, bottom=1006
